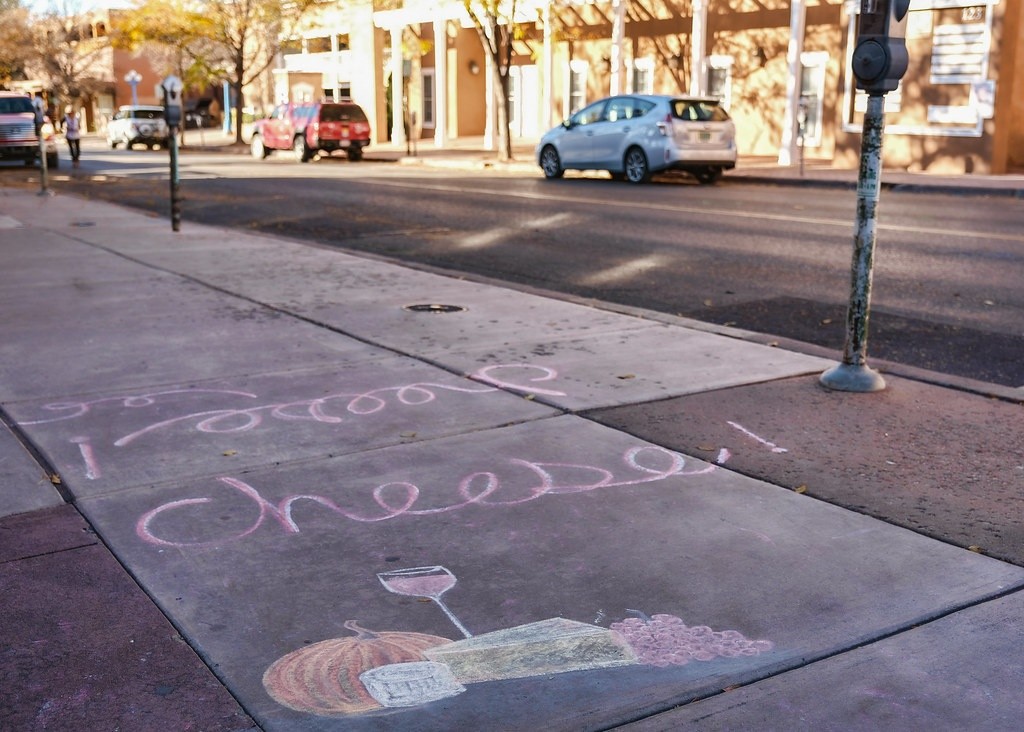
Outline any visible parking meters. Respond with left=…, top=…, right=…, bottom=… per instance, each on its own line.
left=820, top=1, right=912, bottom=392
left=31, top=97, right=54, bottom=197
left=160, top=73, right=184, bottom=233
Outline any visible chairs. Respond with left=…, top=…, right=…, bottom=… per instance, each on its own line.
left=617, top=109, right=627, bottom=121
left=631, top=109, right=642, bottom=118
left=680, top=109, right=690, bottom=119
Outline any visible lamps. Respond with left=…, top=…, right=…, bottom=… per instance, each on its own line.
left=753, top=47, right=762, bottom=66
left=602, top=57, right=610, bottom=72
left=671, top=54, right=680, bottom=68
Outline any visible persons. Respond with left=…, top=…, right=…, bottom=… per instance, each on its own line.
left=58, top=110, right=84, bottom=164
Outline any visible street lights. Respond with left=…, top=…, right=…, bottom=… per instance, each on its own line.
left=212, top=60, right=236, bottom=137
left=123, top=69, right=142, bottom=106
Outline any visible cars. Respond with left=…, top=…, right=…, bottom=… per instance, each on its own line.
left=534, top=92, right=737, bottom=184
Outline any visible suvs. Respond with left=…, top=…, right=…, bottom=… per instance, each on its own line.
left=109, top=105, right=172, bottom=153
left=0, top=90, right=58, bottom=172
left=251, top=101, right=373, bottom=163
